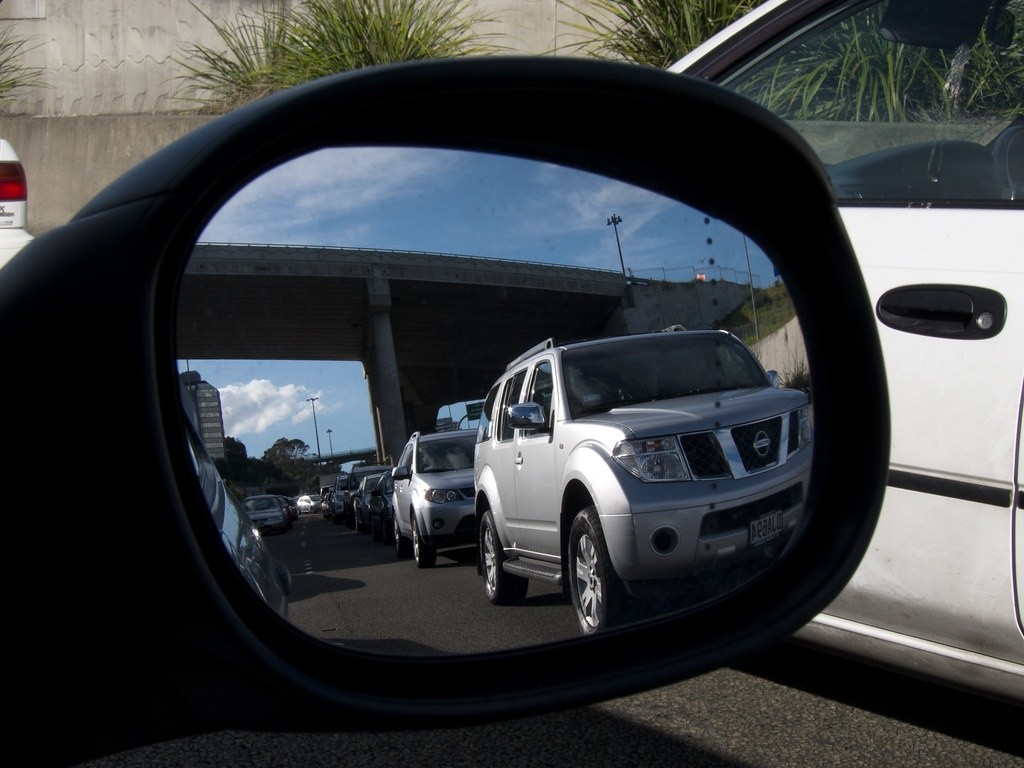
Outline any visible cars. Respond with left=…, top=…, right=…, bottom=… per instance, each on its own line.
left=664, top=0, right=1024, bottom=714
left=369, top=470, right=394, bottom=547
left=350, top=472, right=383, bottom=535
left=320, top=485, right=334, bottom=518
left=334, top=474, right=350, bottom=525
left=279, top=497, right=298, bottom=520
left=240, top=495, right=288, bottom=535
left=296, top=495, right=322, bottom=514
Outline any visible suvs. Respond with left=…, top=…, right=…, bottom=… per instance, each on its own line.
left=389, top=429, right=481, bottom=569
left=472, top=326, right=814, bottom=637
